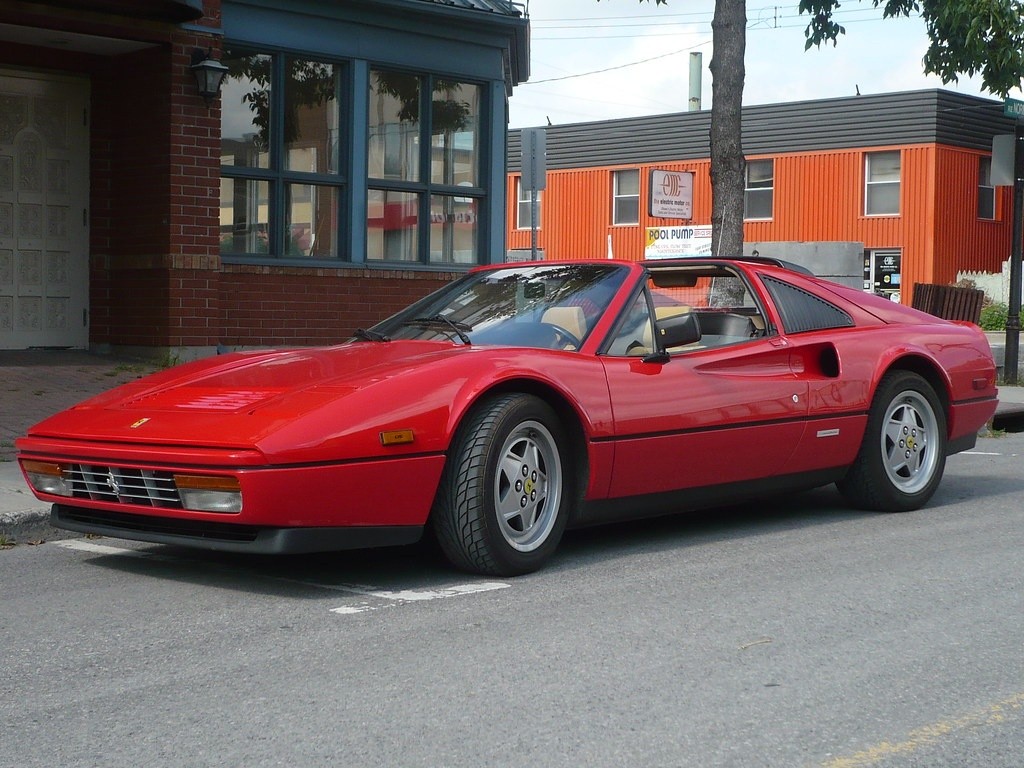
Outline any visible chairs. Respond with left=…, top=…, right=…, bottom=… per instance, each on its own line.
left=628, top=305, right=704, bottom=354
left=540, top=306, right=586, bottom=349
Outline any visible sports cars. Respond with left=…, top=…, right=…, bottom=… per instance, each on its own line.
left=14, top=253, right=1000, bottom=579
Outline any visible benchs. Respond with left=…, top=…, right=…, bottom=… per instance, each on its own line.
left=655, top=312, right=764, bottom=346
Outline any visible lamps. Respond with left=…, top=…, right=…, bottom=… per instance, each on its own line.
left=191, top=47, right=229, bottom=110
